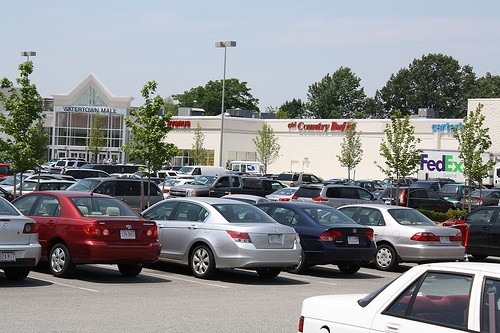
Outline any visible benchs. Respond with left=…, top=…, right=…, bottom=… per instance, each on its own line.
left=222, top=211, right=256, bottom=222
left=78, top=206, right=120, bottom=216
left=400, top=295, right=469, bottom=325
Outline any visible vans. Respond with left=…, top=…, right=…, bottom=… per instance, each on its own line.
left=226, top=160, right=266, bottom=174
left=177, top=165, right=226, bottom=177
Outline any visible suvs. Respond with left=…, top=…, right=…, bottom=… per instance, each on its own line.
left=278, top=172, right=324, bottom=187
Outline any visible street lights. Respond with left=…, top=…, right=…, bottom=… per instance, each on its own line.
left=215, top=41, right=237, bottom=166
left=20, top=51, right=37, bottom=136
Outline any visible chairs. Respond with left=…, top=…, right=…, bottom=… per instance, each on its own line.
left=188, top=209, right=198, bottom=220
left=272, top=212, right=288, bottom=224
left=47, top=204, right=59, bottom=216
left=358, top=215, right=370, bottom=225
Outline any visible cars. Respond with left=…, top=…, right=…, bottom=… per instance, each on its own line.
left=64, top=163, right=217, bottom=214
left=138, top=194, right=377, bottom=280
left=0, top=157, right=146, bottom=204
left=299, top=262, right=500, bottom=333
left=438, top=205, right=500, bottom=258
left=222, top=173, right=384, bottom=208
left=325, top=177, right=500, bottom=212
left=0, top=190, right=162, bottom=280
left=319, top=204, right=465, bottom=275
